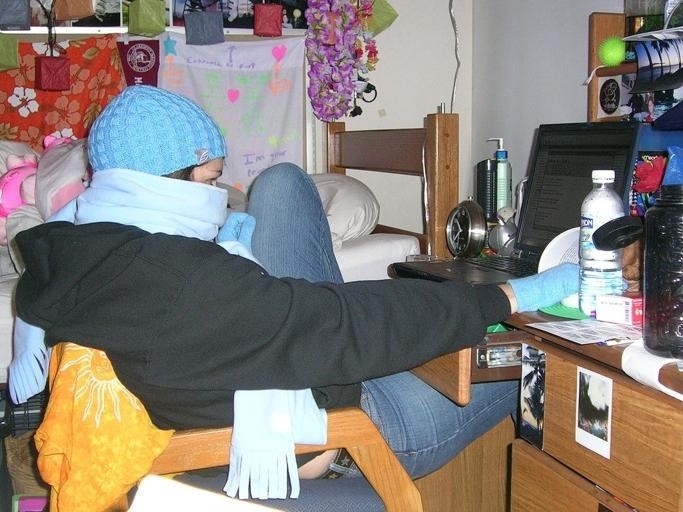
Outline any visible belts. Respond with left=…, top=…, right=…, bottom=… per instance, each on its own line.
left=320, top=448, right=354, bottom=480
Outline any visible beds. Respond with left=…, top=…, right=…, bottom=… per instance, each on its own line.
left=1, top=109, right=462, bottom=394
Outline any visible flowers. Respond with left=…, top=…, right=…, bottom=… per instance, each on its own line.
left=302, top=0, right=380, bottom=125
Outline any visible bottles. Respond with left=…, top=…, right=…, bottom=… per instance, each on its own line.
left=578, top=169, right=625, bottom=320
left=641, top=185, right=682, bottom=359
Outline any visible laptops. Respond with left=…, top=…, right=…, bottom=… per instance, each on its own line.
left=388, top=122, right=642, bottom=288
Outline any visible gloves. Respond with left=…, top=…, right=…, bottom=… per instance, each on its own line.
left=507, top=262, right=580, bottom=314
left=215, top=212, right=256, bottom=252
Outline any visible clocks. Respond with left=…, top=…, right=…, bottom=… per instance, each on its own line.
left=444, top=200, right=489, bottom=260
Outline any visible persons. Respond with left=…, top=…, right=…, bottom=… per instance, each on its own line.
left=14, top=83, right=583, bottom=487
left=645, top=98, right=655, bottom=121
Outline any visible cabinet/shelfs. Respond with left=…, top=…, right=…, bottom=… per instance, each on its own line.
left=385, top=248, right=683, bottom=512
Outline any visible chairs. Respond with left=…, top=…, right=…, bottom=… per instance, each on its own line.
left=44, top=329, right=425, bottom=512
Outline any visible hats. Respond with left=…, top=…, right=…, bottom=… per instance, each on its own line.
left=87, top=84, right=228, bottom=177
